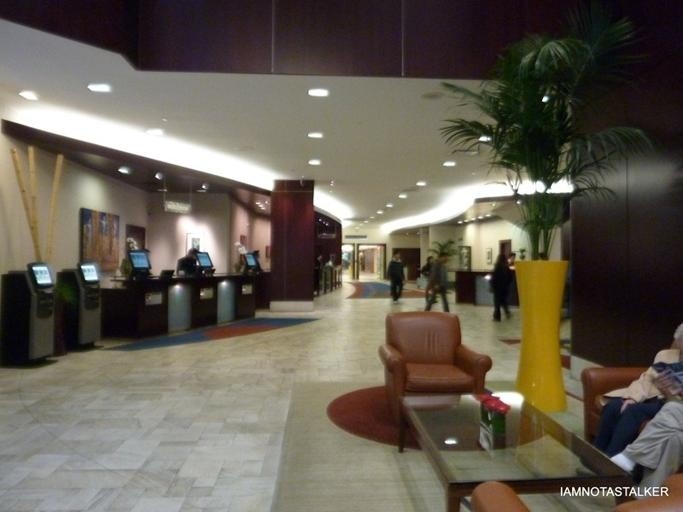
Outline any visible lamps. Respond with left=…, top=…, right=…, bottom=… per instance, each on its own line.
left=161, top=175, right=193, bottom=214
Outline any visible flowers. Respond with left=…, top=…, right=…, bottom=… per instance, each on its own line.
left=473, top=390, right=511, bottom=413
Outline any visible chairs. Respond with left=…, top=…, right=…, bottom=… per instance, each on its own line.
left=580, top=367, right=655, bottom=444
left=469, top=474, right=683, bottom=512
left=378, top=311, right=493, bottom=426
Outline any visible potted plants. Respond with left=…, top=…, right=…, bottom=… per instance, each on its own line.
left=436, top=18, right=656, bottom=413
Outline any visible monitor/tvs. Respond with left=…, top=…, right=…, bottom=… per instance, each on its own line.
left=195, top=252, right=213, bottom=267
left=29, top=262, right=54, bottom=289
left=244, top=253, right=258, bottom=267
left=128, top=250, right=151, bottom=270
left=78, top=261, right=100, bottom=284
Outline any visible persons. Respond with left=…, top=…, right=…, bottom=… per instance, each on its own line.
left=176, top=247, right=201, bottom=275
left=575, top=401, right=683, bottom=499
left=314, top=254, right=322, bottom=290
left=421, top=256, right=438, bottom=304
left=387, top=251, right=406, bottom=304
left=507, top=252, right=517, bottom=265
left=423, top=251, right=450, bottom=312
left=588, top=323, right=683, bottom=458
left=489, top=253, right=515, bottom=322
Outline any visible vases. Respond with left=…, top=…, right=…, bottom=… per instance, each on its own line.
left=479, top=404, right=506, bottom=436
left=478, top=424, right=505, bottom=456
